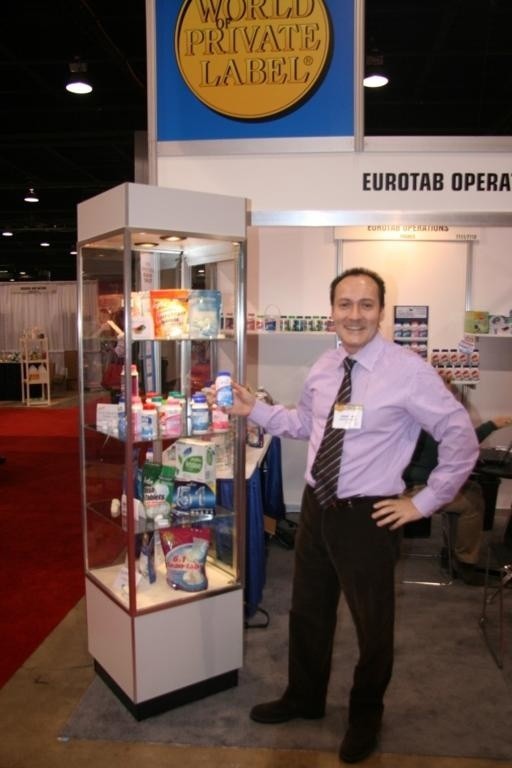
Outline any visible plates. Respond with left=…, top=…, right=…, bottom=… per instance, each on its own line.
left=19, top=327, right=50, bottom=408
left=74, top=180, right=246, bottom=706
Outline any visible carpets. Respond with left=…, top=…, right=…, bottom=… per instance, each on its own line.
left=310, top=357, right=357, bottom=510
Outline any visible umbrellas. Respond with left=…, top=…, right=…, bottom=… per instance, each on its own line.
left=251, top=699, right=325, bottom=723
left=340, top=726, right=378, bottom=762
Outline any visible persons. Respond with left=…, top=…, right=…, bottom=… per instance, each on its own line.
left=208, top=266, right=481, bottom=765
left=401, top=375, right=512, bottom=587
left=110, top=307, right=144, bottom=404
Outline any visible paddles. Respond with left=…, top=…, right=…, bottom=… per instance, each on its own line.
left=60, top=511, right=511, bottom=760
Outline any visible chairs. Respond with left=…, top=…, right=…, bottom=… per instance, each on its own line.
left=443, top=556, right=503, bottom=584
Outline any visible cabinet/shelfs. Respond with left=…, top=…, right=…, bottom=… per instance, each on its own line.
left=393, top=321, right=480, bottom=381
left=121, top=363, right=231, bottom=437
left=221, top=312, right=232, bottom=328
left=247, top=313, right=334, bottom=331
